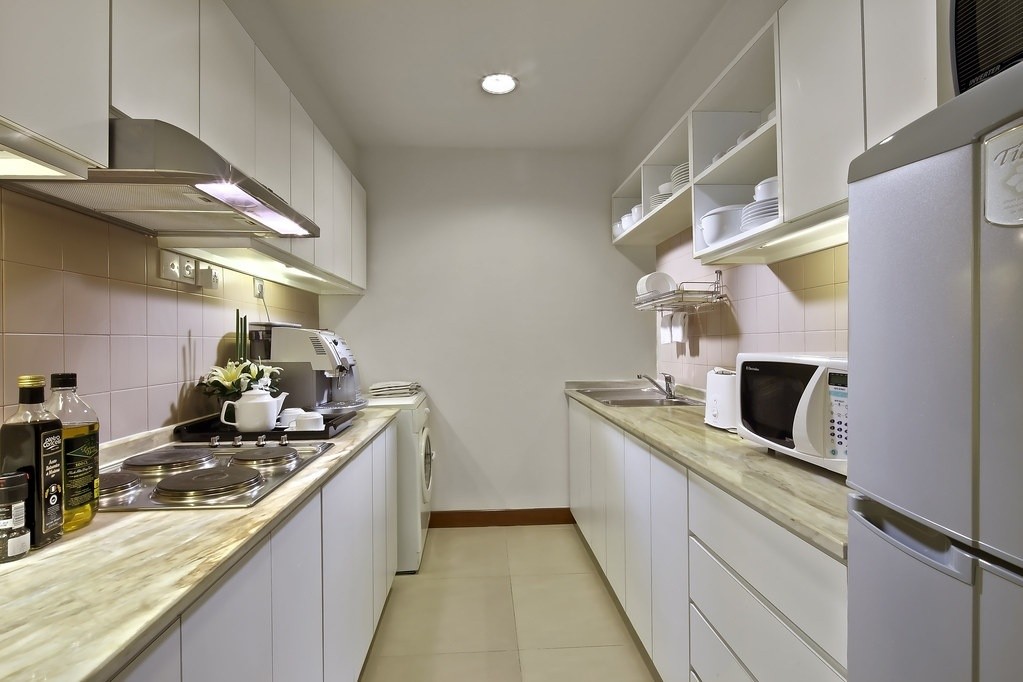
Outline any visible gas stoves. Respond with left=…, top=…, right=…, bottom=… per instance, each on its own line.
left=82, top=435, right=334, bottom=509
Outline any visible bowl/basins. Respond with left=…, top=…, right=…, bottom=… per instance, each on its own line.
left=631, top=204, right=642, bottom=221
left=620, top=214, right=634, bottom=232
left=611, top=220, right=622, bottom=238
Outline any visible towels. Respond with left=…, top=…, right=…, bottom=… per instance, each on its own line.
left=660, top=311, right=689, bottom=344
left=368, top=381, right=422, bottom=399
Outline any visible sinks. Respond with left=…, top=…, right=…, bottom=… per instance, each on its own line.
left=602, top=400, right=702, bottom=407
left=578, top=389, right=668, bottom=400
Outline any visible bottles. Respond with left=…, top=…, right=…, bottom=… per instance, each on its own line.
left=1, top=375, right=65, bottom=548
left=46, top=372, right=99, bottom=533
left=0, top=471, right=31, bottom=563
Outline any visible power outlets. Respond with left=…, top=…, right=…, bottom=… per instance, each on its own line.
left=201, top=269, right=220, bottom=289
left=253, top=277, right=264, bottom=298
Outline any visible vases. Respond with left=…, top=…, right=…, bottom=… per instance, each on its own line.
left=218, top=395, right=238, bottom=431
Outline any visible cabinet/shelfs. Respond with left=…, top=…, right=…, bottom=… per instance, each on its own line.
left=570, top=396, right=849, bottom=682
left=0, top=0, right=368, bottom=296
left=612, top=0, right=949, bottom=267
left=107, top=415, right=397, bottom=682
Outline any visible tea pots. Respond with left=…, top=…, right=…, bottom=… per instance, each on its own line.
left=221, top=385, right=289, bottom=433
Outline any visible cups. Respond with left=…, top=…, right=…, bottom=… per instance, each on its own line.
left=288, top=411, right=325, bottom=431
left=275, top=407, right=306, bottom=427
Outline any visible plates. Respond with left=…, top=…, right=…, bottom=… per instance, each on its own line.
left=636, top=272, right=677, bottom=296
left=646, top=104, right=781, bottom=247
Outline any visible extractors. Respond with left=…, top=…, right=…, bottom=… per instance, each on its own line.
left=3, top=116, right=321, bottom=250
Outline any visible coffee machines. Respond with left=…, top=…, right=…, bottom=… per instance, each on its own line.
left=246, top=319, right=369, bottom=412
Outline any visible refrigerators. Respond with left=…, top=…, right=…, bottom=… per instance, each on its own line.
left=845, top=51, right=1023, bottom=682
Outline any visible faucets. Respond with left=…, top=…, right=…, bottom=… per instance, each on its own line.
left=636, top=371, right=676, bottom=400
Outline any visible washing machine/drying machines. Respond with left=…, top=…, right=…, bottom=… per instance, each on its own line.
left=361, top=391, right=437, bottom=578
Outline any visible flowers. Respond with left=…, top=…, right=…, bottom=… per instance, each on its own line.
left=193, top=357, right=282, bottom=400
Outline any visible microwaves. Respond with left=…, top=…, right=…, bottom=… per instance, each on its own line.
left=735, top=352, right=848, bottom=481
